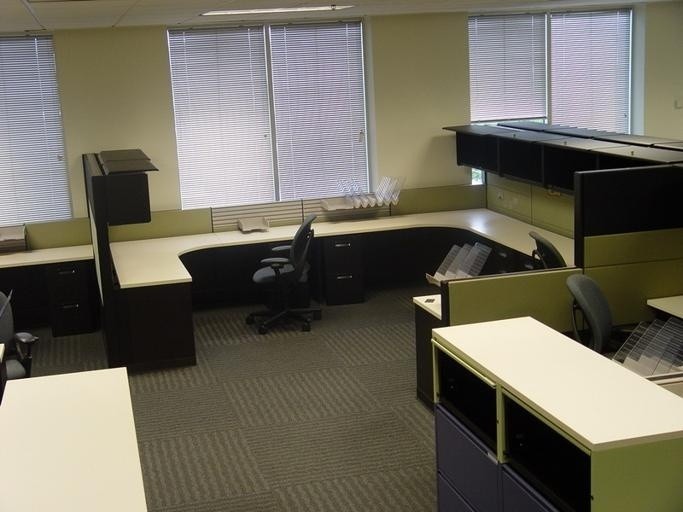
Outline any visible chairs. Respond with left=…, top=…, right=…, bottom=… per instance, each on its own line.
left=244, top=212, right=325, bottom=337
left=0, top=290, right=40, bottom=402
left=566, top=272, right=682, bottom=375
left=527, top=230, right=568, bottom=271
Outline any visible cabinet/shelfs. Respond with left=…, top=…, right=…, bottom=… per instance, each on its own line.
left=438, top=122, right=682, bottom=235
left=318, top=236, right=363, bottom=306
left=431, top=314, right=682, bottom=511
left=80, top=147, right=160, bottom=225
left=48, top=261, right=88, bottom=338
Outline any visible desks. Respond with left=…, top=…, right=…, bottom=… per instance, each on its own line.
left=106, top=207, right=575, bottom=414
left=0, top=363, right=148, bottom=512
left=0, top=244, right=96, bottom=338
left=646, top=295, right=682, bottom=322
